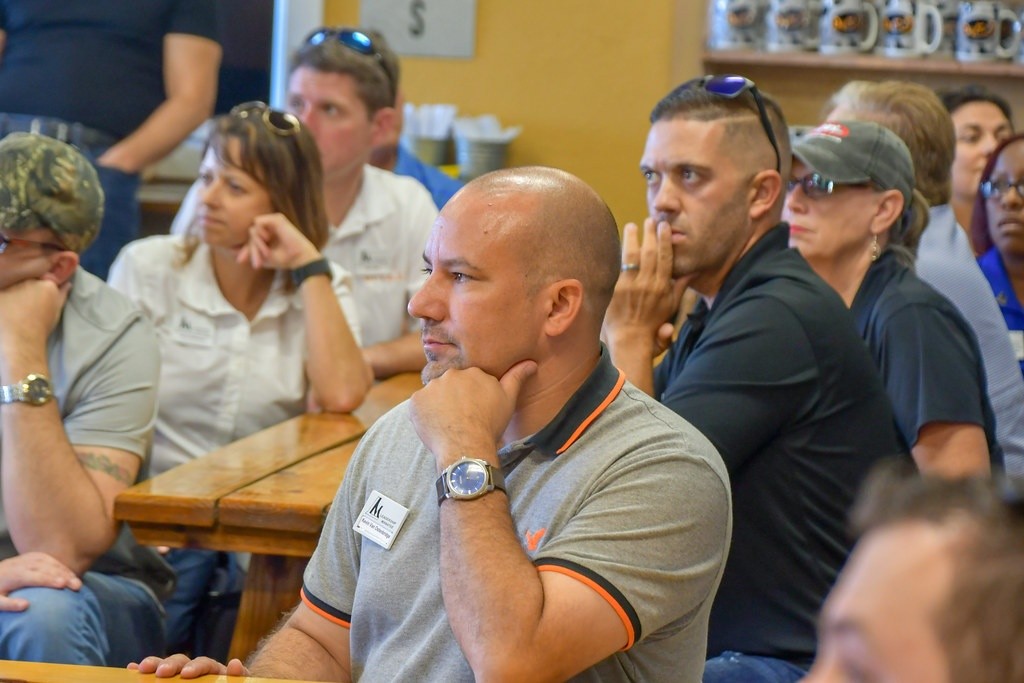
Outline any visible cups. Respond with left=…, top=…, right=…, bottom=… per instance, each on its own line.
left=704, top=1, right=1024, bottom=69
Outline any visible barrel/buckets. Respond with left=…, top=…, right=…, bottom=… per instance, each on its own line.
left=398, top=136, right=450, bottom=165
left=453, top=134, right=507, bottom=180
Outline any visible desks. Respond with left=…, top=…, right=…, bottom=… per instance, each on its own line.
left=110, top=370, right=421, bottom=666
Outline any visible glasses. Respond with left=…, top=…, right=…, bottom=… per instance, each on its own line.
left=304, top=25, right=395, bottom=105
left=0, top=233, right=66, bottom=257
left=682, top=73, right=782, bottom=177
left=786, top=169, right=885, bottom=202
left=232, top=101, right=304, bottom=175
left=978, top=179, right=1024, bottom=199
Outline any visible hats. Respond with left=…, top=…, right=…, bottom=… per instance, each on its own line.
left=790, top=118, right=913, bottom=205
left=0, top=131, right=105, bottom=255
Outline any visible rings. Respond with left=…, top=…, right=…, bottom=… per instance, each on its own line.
left=622, top=264, right=639, bottom=270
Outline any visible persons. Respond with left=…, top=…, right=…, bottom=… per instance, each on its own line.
left=784, top=121, right=1006, bottom=486
left=969, top=132, right=1024, bottom=372
left=106, top=100, right=374, bottom=659
left=602, top=74, right=922, bottom=683
left=127, top=166, right=733, bottom=683
left=826, top=80, right=1024, bottom=466
left=0, top=0, right=221, bottom=285
left=938, top=89, right=1016, bottom=258
left=796, top=469, right=1024, bottom=683
left=171, top=25, right=439, bottom=381
left=0, top=130, right=161, bottom=669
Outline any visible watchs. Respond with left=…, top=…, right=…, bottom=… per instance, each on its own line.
left=0, top=373, right=52, bottom=405
left=435, top=456, right=508, bottom=508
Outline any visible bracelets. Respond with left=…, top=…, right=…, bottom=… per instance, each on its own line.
left=292, top=258, right=333, bottom=287
